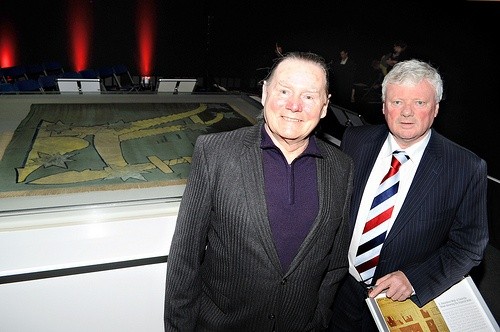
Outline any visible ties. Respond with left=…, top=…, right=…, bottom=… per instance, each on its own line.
left=353, top=150, right=409, bottom=288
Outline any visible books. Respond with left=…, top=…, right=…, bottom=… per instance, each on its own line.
left=365, top=275, right=500, bottom=332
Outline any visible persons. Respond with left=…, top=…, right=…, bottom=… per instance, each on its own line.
left=162, top=43, right=354, bottom=332
left=253, top=41, right=417, bottom=108
left=330, top=58, right=488, bottom=332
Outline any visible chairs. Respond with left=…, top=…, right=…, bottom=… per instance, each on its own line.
left=0, top=61, right=144, bottom=93
